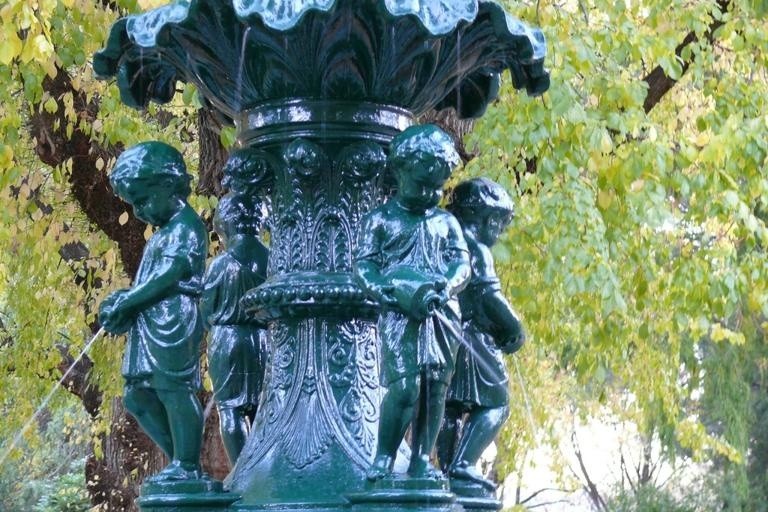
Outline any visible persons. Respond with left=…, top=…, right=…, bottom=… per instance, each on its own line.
left=433, top=177, right=526, bottom=492
left=349, top=124, right=473, bottom=480
left=94, top=141, right=207, bottom=480
left=203, top=183, right=270, bottom=461
